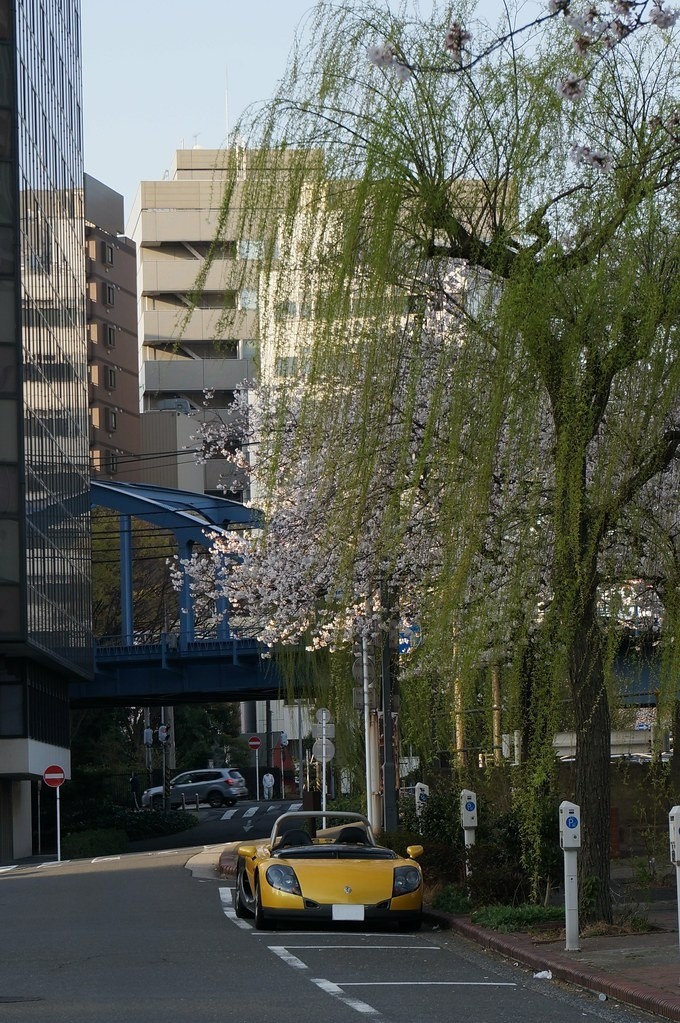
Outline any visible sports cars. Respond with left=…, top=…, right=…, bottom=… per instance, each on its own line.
left=232, top=810, right=427, bottom=933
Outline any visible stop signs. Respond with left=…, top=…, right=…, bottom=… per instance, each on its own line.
left=42, top=763, right=66, bottom=788
left=248, top=737, right=261, bottom=750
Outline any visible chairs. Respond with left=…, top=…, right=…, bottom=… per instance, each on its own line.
left=276, top=829, right=313, bottom=848
left=334, top=827, right=371, bottom=843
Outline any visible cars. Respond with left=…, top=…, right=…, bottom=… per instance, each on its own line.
left=559, top=750, right=674, bottom=764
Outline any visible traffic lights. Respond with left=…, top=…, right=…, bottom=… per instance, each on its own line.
left=157, top=722, right=170, bottom=742
left=143, top=728, right=155, bottom=748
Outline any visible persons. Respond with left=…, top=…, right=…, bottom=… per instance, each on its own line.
left=262, top=770, right=274, bottom=800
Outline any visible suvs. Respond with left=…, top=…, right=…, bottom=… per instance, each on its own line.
left=141, top=768, right=249, bottom=811
